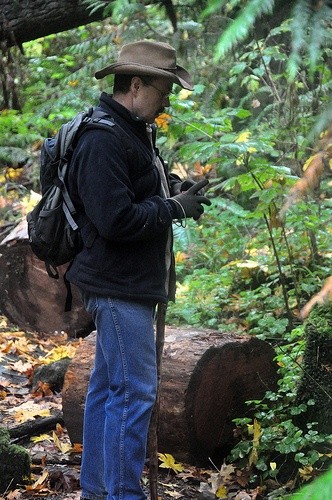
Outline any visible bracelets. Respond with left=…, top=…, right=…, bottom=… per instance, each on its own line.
left=166, top=197, right=187, bottom=227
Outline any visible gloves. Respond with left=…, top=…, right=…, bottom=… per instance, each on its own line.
left=166, top=178, right=212, bottom=221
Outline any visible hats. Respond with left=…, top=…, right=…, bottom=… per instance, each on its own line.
left=94, top=41, right=195, bottom=91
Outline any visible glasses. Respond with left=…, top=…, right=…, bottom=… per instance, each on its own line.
left=141, top=79, right=171, bottom=99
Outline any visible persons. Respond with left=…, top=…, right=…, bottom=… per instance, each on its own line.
left=65, top=40, right=212, bottom=500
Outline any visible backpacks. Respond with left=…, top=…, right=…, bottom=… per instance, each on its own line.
left=26, top=107, right=171, bottom=312
